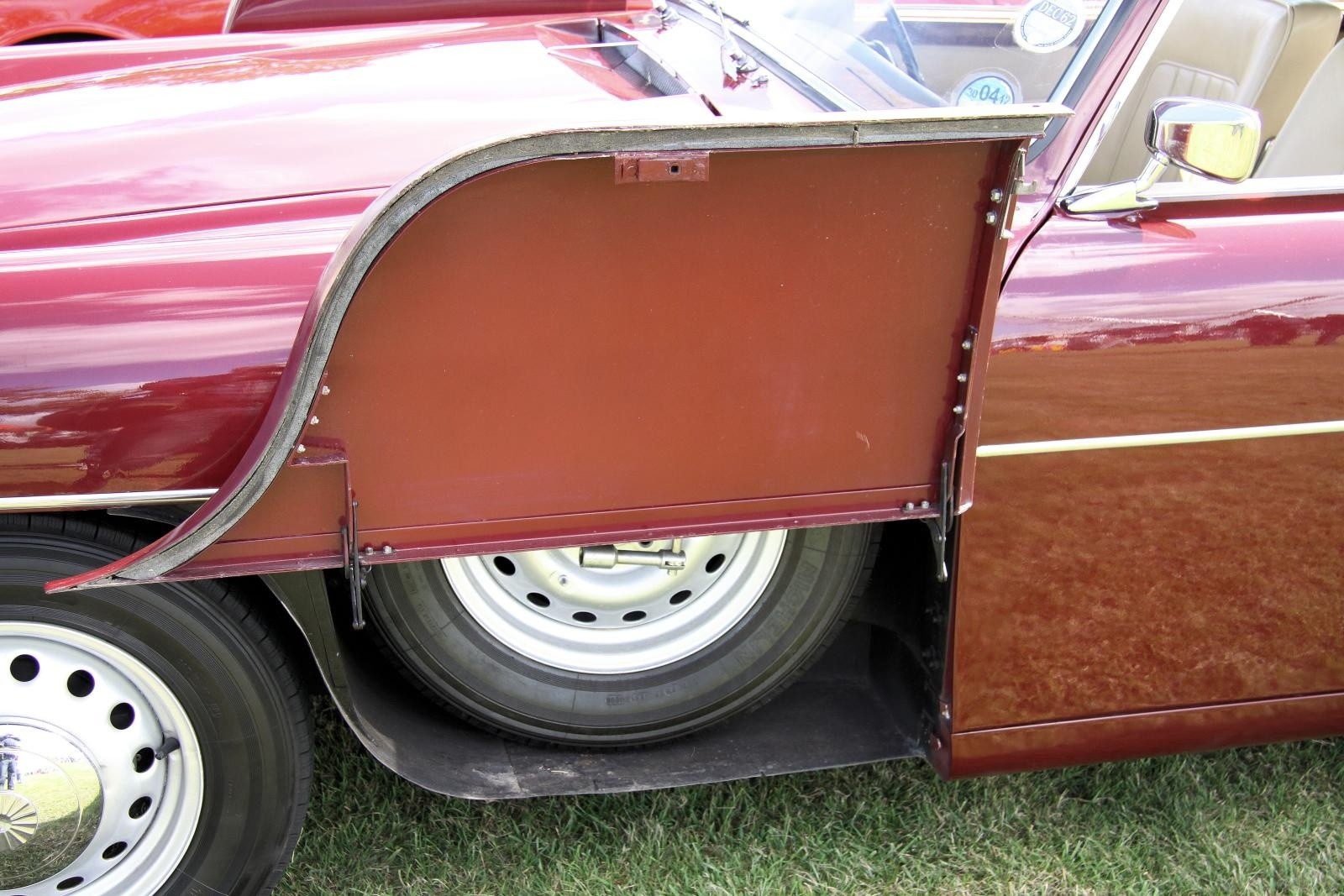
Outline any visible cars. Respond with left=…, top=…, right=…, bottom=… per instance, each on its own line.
left=0, top=2, right=1342, bottom=896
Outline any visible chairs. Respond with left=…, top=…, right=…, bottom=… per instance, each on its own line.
left=1075, top=1, right=1344, bottom=181
